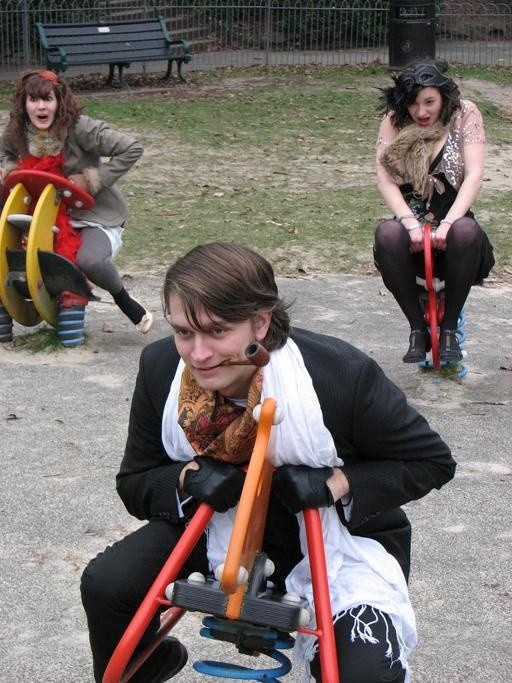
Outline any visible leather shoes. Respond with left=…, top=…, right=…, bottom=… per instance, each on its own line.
left=135, top=309, right=153, bottom=335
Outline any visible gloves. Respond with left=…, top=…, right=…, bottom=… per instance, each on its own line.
left=269, top=462, right=337, bottom=514
left=182, top=453, right=248, bottom=514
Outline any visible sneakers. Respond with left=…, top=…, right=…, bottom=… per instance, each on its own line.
left=402, top=326, right=433, bottom=364
left=438, top=328, right=464, bottom=361
left=124, top=636, right=189, bottom=681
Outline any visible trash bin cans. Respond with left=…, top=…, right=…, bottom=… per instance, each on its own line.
left=387, top=0, right=437, bottom=72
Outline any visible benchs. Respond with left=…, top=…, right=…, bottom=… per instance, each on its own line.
left=33, top=15, right=192, bottom=91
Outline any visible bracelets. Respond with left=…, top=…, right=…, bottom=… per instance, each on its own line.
left=397, top=215, right=419, bottom=223
left=440, top=219, right=453, bottom=225
left=406, top=223, right=422, bottom=234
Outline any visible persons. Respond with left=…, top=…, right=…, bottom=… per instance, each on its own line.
left=0, top=71, right=153, bottom=334
left=372, top=54, right=496, bottom=362
left=80, top=243, right=455, bottom=683
